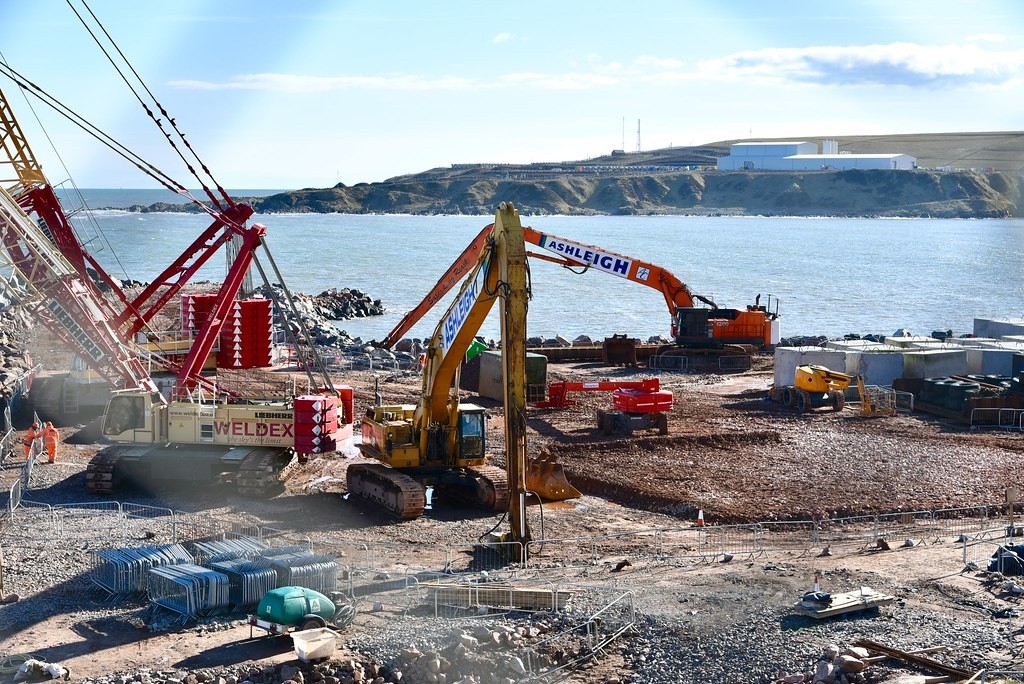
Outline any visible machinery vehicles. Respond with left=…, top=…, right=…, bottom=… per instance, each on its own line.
left=343, top=200, right=582, bottom=557
left=535, top=376, right=675, bottom=434
left=0, top=86, right=354, bottom=495
left=376, top=220, right=780, bottom=374
left=768, top=363, right=878, bottom=416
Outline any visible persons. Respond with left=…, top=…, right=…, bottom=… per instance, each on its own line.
left=24, top=422, right=39, bottom=459
left=36, top=421, right=59, bottom=463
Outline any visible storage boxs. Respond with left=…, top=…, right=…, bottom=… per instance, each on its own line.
left=289, top=627, right=340, bottom=660
left=774, top=318, right=1024, bottom=399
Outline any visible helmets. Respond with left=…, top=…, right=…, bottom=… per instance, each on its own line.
left=33, top=423, right=39, bottom=429
left=47, top=421, right=54, bottom=426
